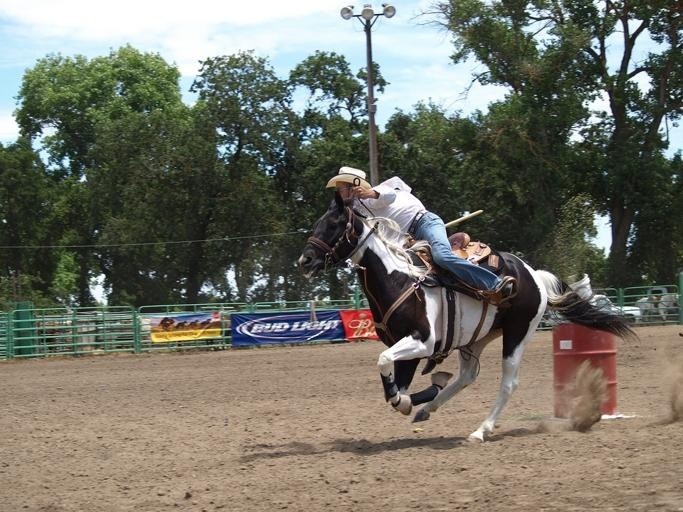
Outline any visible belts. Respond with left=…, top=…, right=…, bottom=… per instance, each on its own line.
left=407, top=209, right=429, bottom=234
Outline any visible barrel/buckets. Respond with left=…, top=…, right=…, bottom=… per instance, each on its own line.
left=568, top=273, right=597, bottom=306
left=553, top=322, right=618, bottom=419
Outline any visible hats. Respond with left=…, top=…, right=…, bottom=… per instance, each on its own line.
left=325, top=166, right=373, bottom=190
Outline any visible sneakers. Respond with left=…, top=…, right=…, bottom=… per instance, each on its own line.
left=497, top=282, right=515, bottom=297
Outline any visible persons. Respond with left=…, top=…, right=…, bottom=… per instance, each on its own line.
left=326, top=166, right=513, bottom=300
left=360, top=294, right=370, bottom=308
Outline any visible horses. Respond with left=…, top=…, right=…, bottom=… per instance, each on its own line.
left=634, top=293, right=678, bottom=326
left=295, top=188, right=642, bottom=444
